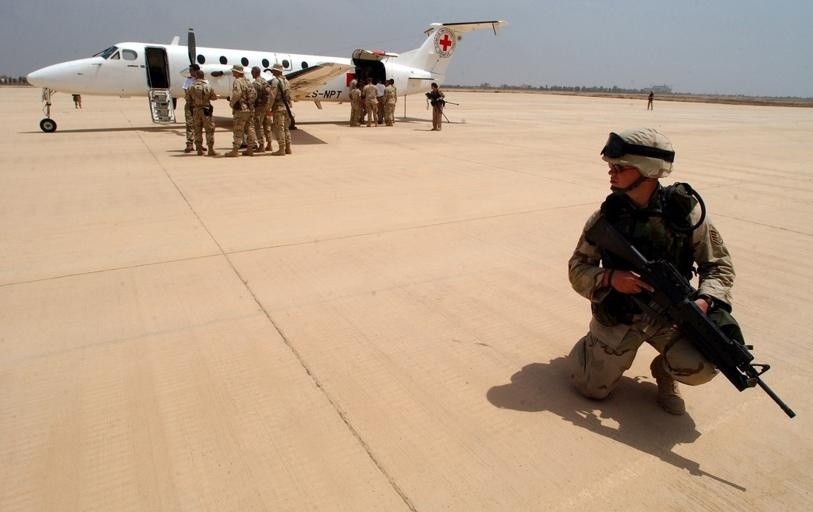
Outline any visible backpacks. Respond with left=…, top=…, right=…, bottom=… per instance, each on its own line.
left=253, top=80, right=270, bottom=105
left=238, top=78, right=257, bottom=105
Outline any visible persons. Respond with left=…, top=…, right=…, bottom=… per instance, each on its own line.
left=606, top=268, right=616, bottom=287
left=223, top=64, right=256, bottom=158
left=645, top=90, right=655, bottom=110
left=71, top=94, right=81, bottom=110
left=188, top=70, right=218, bottom=156
left=250, top=66, right=274, bottom=153
left=347, top=77, right=399, bottom=128
left=183, top=64, right=207, bottom=151
left=427, top=83, right=446, bottom=132
left=264, top=63, right=293, bottom=157
left=567, top=126, right=737, bottom=416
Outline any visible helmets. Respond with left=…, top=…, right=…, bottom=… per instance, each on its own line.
left=603, top=127, right=675, bottom=179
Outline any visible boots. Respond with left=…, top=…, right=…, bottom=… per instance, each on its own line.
left=223, top=141, right=290, bottom=157
left=184, top=143, right=216, bottom=157
left=649, top=354, right=686, bottom=415
left=349, top=118, right=395, bottom=127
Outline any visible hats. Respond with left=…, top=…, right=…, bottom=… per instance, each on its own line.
left=231, top=65, right=244, bottom=75
left=271, top=64, right=282, bottom=73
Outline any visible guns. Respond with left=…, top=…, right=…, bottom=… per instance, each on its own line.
left=585, top=217, right=796, bottom=418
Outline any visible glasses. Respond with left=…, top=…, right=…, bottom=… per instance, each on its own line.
left=602, top=132, right=629, bottom=159
left=608, top=162, right=636, bottom=172
left=189, top=69, right=192, bottom=71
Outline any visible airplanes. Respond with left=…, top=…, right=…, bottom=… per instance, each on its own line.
left=21, top=19, right=508, bottom=132
